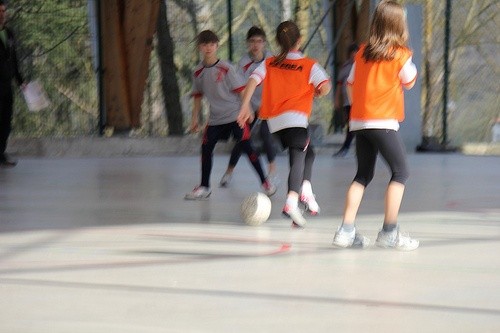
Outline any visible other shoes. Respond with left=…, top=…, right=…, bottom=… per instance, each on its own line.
left=1, top=154, right=18, bottom=167
left=219, top=174, right=233, bottom=187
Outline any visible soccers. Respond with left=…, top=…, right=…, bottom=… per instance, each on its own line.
left=239, top=192, right=272, bottom=228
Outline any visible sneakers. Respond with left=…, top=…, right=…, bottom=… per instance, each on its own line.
left=374, top=230, right=420, bottom=252
left=261, top=182, right=275, bottom=195
left=186, top=186, right=211, bottom=201
left=299, top=192, right=322, bottom=215
left=280, top=203, right=304, bottom=227
left=333, top=225, right=369, bottom=250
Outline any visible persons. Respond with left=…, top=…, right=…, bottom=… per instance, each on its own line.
left=237, top=20, right=331, bottom=227
left=183, top=29, right=277, bottom=200
left=220, top=26, right=279, bottom=187
left=332, top=45, right=358, bottom=157
left=0, top=4, right=26, bottom=168
left=332, top=0, right=419, bottom=250
